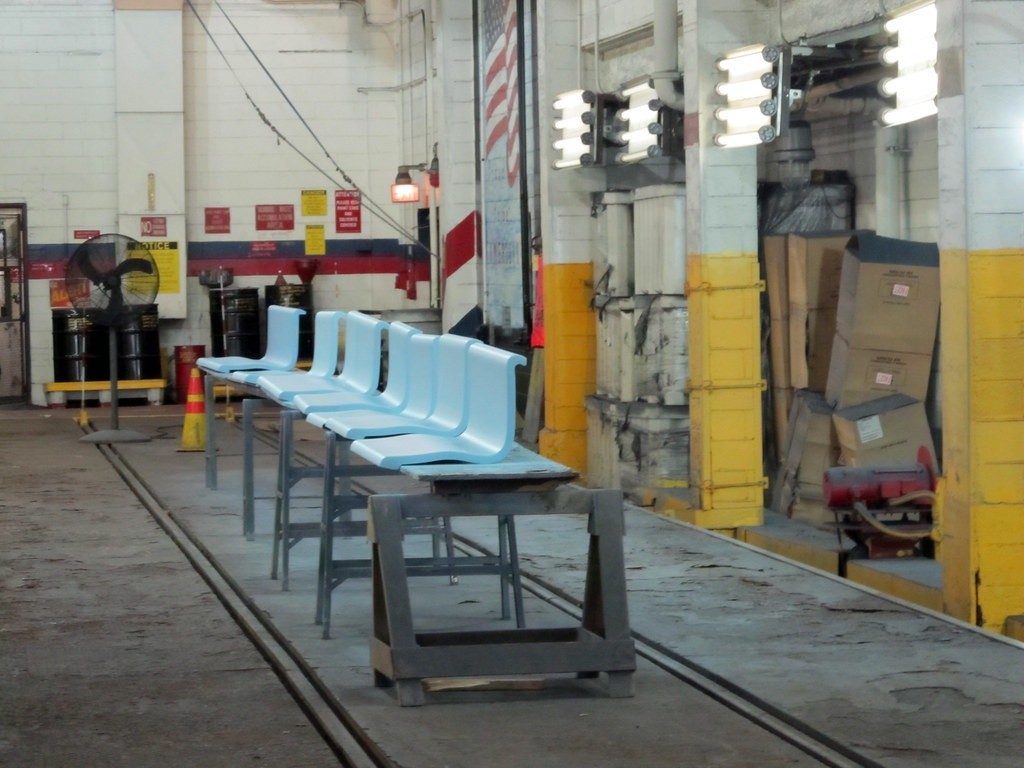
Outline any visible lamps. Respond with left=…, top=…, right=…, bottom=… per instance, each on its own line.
left=714, top=43, right=780, bottom=148
left=390, top=142, right=439, bottom=203
left=879, top=0, right=939, bottom=127
left=552, top=89, right=596, bottom=170
left=616, top=75, right=665, bottom=163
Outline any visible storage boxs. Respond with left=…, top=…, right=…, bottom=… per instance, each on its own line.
left=764, top=229, right=941, bottom=522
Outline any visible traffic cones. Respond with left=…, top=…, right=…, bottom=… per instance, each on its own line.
left=174, top=367, right=220, bottom=453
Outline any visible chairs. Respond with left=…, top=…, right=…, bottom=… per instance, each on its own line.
left=195, top=305, right=579, bottom=641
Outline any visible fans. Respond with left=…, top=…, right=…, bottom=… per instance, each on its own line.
left=65, top=234, right=160, bottom=444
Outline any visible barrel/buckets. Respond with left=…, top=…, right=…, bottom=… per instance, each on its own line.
left=266, top=284, right=315, bottom=360
left=51, top=308, right=110, bottom=409
left=160, top=348, right=170, bottom=383
left=175, top=345, right=206, bottom=403
left=116, top=304, right=163, bottom=407
left=208, top=287, right=260, bottom=359
